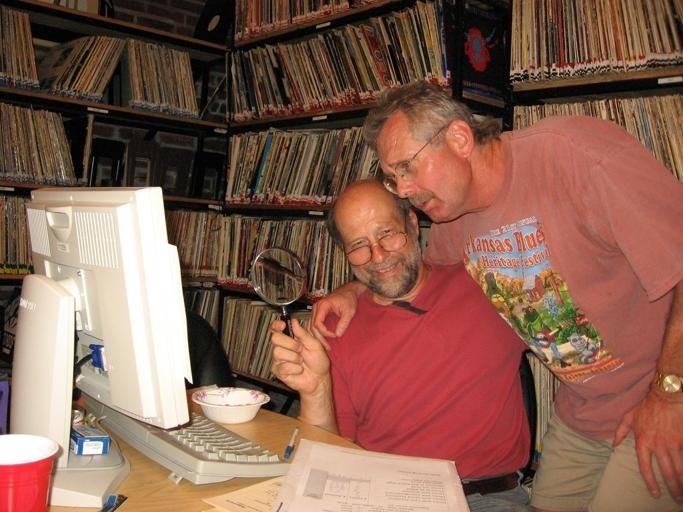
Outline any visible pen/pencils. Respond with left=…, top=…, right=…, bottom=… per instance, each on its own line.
left=284, top=428, right=299, bottom=458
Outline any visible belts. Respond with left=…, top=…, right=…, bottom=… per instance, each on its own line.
left=462, top=460, right=532, bottom=496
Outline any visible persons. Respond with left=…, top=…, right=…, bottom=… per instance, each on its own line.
left=270, top=178, right=536, bottom=512
left=309, top=81, right=683, bottom=512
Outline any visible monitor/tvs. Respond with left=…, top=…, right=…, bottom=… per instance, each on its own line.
left=6, top=187, right=191, bottom=509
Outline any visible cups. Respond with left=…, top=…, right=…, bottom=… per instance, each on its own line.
left=0, top=434, right=58, bottom=512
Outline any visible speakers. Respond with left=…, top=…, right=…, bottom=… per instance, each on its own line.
left=9, top=275, right=130, bottom=509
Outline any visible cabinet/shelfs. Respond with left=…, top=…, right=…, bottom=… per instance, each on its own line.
left=219, top=1, right=681, bottom=476
left=1, top=1, right=228, bottom=393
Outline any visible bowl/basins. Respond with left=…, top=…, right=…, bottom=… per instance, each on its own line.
left=191, top=388, right=270, bottom=424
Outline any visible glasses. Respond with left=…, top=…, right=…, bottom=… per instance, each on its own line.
left=382, top=125, right=449, bottom=195
left=343, top=216, right=408, bottom=266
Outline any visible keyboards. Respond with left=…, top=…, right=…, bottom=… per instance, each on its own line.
left=82, top=391, right=291, bottom=485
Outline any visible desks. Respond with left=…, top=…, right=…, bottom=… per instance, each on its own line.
left=44, top=390, right=372, bottom=511
